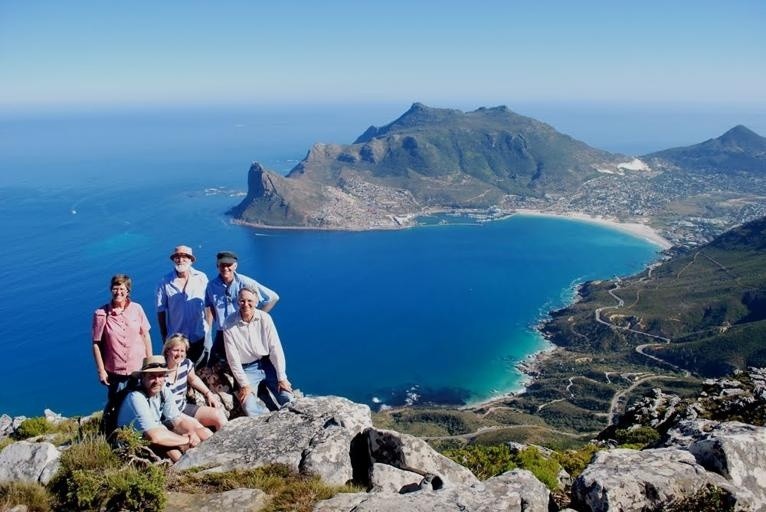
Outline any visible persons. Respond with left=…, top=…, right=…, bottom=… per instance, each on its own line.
left=162, top=332, right=228, bottom=442
left=155, top=245, right=210, bottom=364
left=116, top=354, right=201, bottom=463
left=92, top=275, right=152, bottom=424
left=204, top=252, right=280, bottom=349
left=222, top=287, right=295, bottom=416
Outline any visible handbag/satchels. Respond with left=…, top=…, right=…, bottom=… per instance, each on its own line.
left=206, top=390, right=210, bottom=396
left=188, top=434, right=191, bottom=444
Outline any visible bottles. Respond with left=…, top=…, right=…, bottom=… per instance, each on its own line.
left=217, top=253, right=237, bottom=264
left=170, top=246, right=196, bottom=262
left=132, top=355, right=176, bottom=379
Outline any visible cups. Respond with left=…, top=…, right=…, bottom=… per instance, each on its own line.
left=142, top=363, right=168, bottom=370
